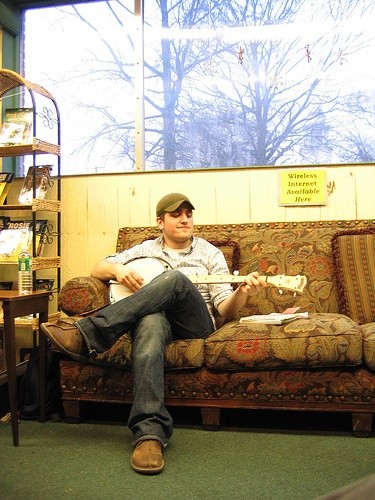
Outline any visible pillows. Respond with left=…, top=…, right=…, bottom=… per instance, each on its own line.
left=140, top=235, right=240, bottom=290
left=331, top=228, right=375, bottom=325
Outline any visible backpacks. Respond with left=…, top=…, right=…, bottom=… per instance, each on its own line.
left=18, top=345, right=59, bottom=420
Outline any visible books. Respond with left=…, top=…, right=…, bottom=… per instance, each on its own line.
left=239, top=313, right=300, bottom=326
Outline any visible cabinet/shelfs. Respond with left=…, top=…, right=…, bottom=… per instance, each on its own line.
left=0, top=68, right=62, bottom=317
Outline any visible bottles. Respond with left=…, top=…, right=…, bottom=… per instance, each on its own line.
left=18, top=246, right=33, bottom=296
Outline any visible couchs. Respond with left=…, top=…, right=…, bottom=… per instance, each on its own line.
left=55, top=219, right=375, bottom=432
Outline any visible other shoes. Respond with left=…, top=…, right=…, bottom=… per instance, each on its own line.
left=40, top=322, right=91, bottom=361
left=130, top=439, right=165, bottom=473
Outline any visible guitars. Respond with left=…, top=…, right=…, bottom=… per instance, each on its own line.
left=108, top=255, right=307, bottom=305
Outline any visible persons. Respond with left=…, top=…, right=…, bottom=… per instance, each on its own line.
left=38, top=193, right=268, bottom=475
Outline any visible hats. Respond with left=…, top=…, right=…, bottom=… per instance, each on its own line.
left=156, top=193, right=195, bottom=217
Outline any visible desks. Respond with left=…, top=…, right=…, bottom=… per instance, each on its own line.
left=0, top=290, right=51, bottom=447
